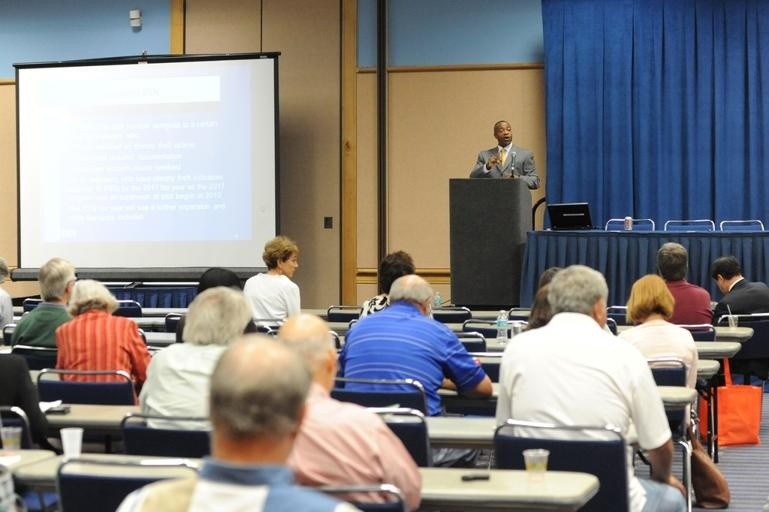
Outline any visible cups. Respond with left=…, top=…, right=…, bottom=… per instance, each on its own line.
left=0, top=426, right=22, bottom=457
left=523, top=447, right=550, bottom=483
left=511, top=323, right=522, bottom=338
left=728, top=315, right=740, bottom=329
left=57, top=427, right=83, bottom=461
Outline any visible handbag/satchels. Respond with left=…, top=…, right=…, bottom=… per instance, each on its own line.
left=699, top=386, right=762, bottom=446
left=689, top=425, right=730, bottom=509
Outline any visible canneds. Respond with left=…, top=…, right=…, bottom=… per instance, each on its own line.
left=472, top=358, right=481, bottom=366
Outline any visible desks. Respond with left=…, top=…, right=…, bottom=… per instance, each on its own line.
left=522, top=230, right=769, bottom=311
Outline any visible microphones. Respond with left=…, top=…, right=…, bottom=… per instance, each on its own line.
left=510, top=151, right=516, bottom=171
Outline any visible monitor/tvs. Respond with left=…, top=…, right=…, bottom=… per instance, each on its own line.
left=547, top=202, right=592, bottom=230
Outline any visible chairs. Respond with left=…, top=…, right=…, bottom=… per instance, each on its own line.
left=720, top=220, right=764, bottom=232
left=665, top=221, right=715, bottom=231
left=605, top=218, right=655, bottom=232
left=1, top=281, right=769, bottom=512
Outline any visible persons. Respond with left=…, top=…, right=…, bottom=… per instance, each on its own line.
left=469, top=121, right=540, bottom=190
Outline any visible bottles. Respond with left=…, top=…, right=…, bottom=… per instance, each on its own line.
left=433, top=291, right=441, bottom=308
left=496, top=309, right=508, bottom=346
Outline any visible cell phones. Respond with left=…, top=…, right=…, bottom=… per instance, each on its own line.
left=46, top=406, right=68, bottom=414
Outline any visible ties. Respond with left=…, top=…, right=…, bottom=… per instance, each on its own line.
left=500, top=149, right=505, bottom=163
